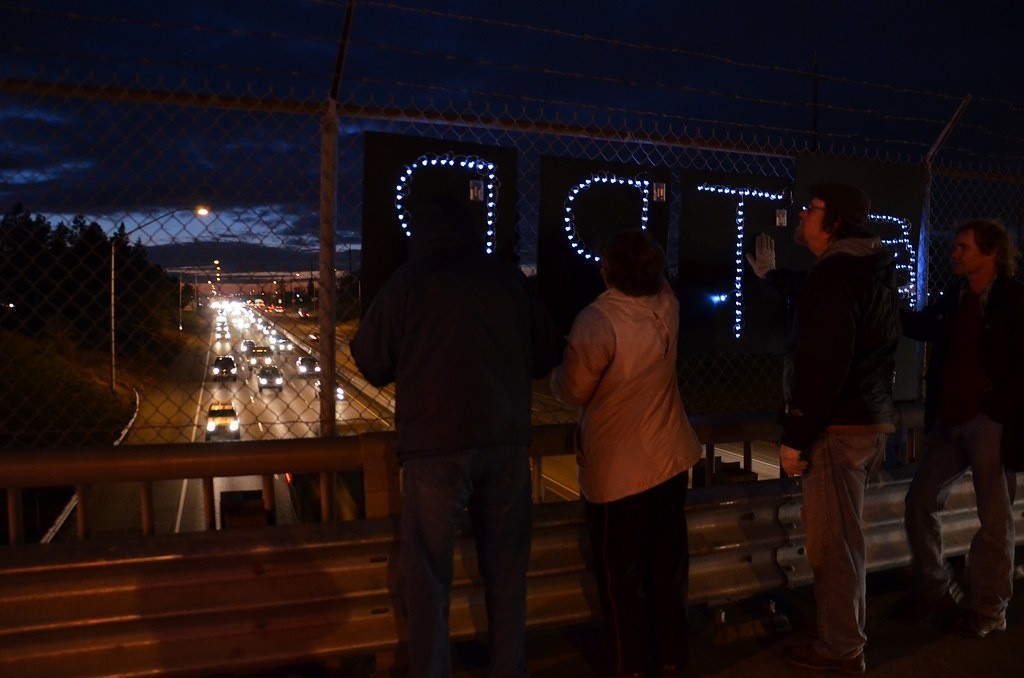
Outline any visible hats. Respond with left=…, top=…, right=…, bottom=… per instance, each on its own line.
left=814, top=183, right=870, bottom=213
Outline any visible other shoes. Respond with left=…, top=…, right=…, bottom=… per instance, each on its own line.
left=909, top=587, right=959, bottom=615
left=958, top=613, right=1007, bottom=637
left=782, top=645, right=866, bottom=674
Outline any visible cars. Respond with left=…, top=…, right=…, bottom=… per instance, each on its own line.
left=204, top=291, right=347, bottom=439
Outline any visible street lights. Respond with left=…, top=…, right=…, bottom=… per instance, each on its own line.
left=110, top=204, right=212, bottom=392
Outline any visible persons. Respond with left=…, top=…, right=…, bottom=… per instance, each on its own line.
left=778, top=182, right=897, bottom=674
left=898, top=219, right=1024, bottom=638
left=351, top=194, right=555, bottom=678
left=545, top=228, right=703, bottom=678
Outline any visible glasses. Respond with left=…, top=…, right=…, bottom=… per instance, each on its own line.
left=804, top=201, right=825, bottom=213
